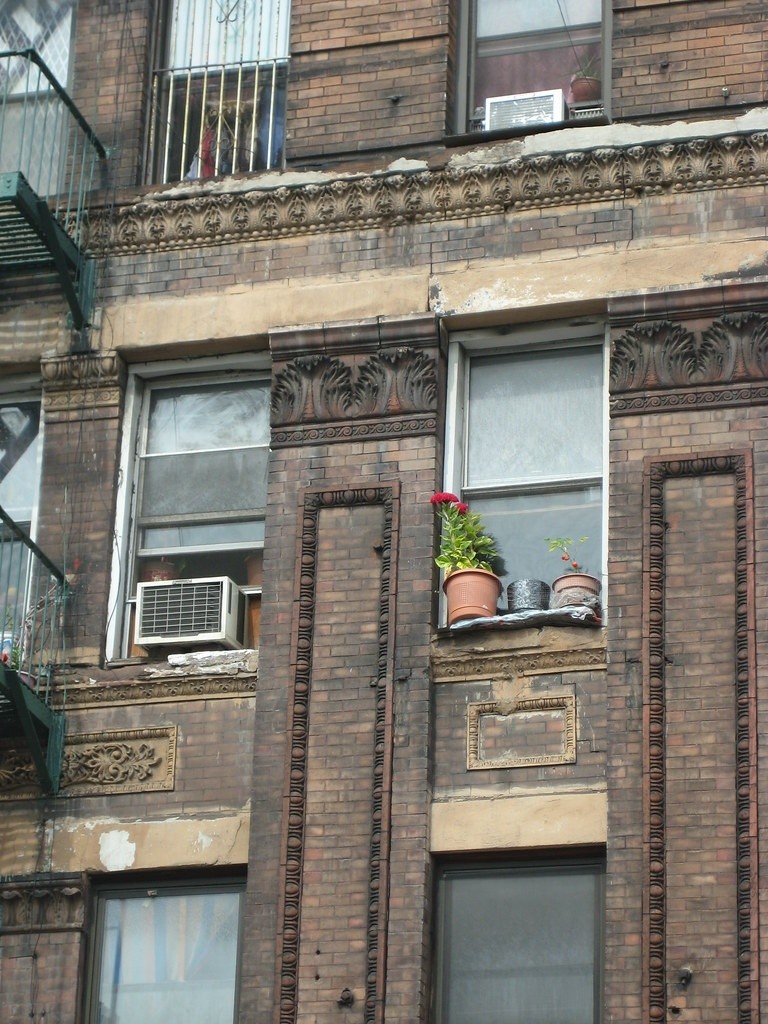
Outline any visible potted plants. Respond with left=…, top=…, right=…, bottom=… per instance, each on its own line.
left=543, top=534, right=603, bottom=613
left=570, top=54, right=601, bottom=103
left=0, top=603, right=20, bottom=669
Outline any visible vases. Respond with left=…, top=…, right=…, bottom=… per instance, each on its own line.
left=443, top=568, right=503, bottom=626
left=15, top=670, right=37, bottom=689
left=507, top=579, right=550, bottom=615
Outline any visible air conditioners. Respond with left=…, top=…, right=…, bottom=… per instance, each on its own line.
left=134, top=576, right=249, bottom=658
left=485, top=88, right=569, bottom=130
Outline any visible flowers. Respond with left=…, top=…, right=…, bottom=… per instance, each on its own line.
left=430, top=491, right=498, bottom=581
